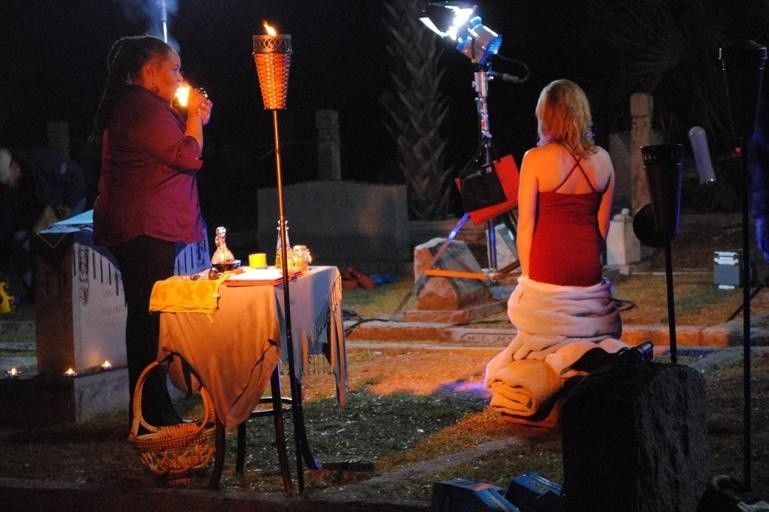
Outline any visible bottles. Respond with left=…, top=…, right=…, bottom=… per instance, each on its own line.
left=275, top=219, right=292, bottom=268
left=618, top=340, right=654, bottom=364
left=210, top=226, right=236, bottom=272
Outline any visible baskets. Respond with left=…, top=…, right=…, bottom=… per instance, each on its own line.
left=127, top=357, right=217, bottom=487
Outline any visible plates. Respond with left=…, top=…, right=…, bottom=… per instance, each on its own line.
left=224, top=268, right=291, bottom=289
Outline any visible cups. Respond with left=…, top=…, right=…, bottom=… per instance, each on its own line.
left=248, top=254, right=267, bottom=268
left=293, top=245, right=311, bottom=272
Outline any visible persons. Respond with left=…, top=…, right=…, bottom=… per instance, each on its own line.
left=0, top=145, right=87, bottom=293
left=502, top=76, right=627, bottom=339
left=91, top=35, right=215, bottom=436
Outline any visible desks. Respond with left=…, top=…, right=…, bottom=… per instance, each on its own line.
left=158, top=264, right=339, bottom=497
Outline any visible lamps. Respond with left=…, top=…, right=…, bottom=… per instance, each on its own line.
left=383, top=6, right=531, bottom=323
